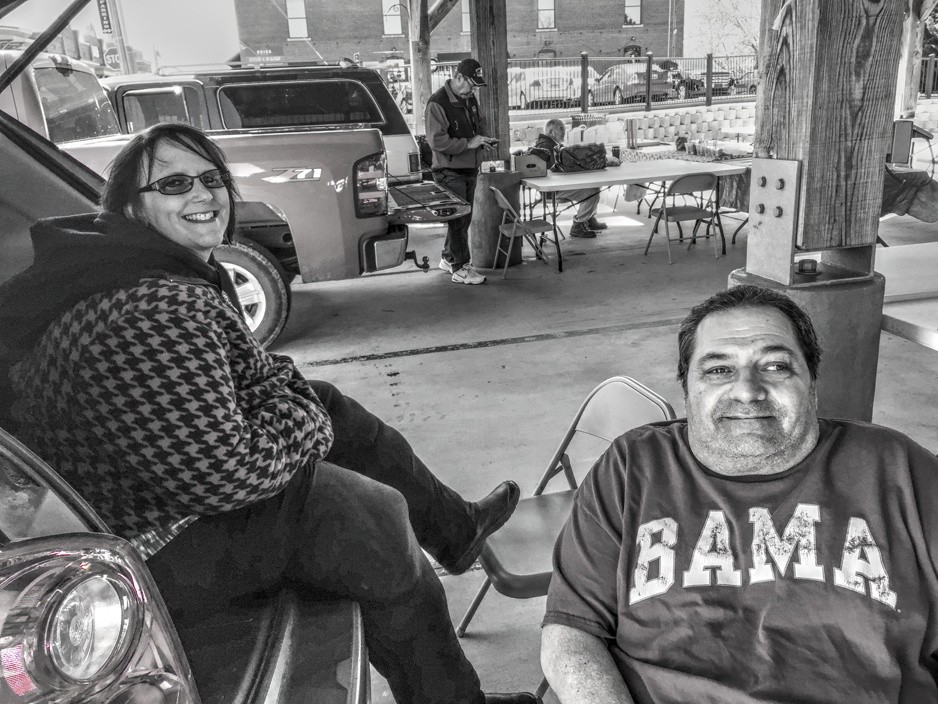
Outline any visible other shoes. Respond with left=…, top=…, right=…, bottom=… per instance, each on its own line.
left=569, top=222, right=595, bottom=238
left=587, top=216, right=607, bottom=230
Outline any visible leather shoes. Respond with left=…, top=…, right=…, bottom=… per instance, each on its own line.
left=449, top=480, right=519, bottom=576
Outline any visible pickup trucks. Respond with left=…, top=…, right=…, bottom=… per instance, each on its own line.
left=1, top=52, right=472, bottom=352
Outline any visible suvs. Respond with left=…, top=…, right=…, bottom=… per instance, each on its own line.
left=97, top=58, right=423, bottom=202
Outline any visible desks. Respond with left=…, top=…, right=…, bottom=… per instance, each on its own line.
left=521, top=159, right=749, bottom=272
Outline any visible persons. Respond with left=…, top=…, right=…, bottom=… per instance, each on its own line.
left=540, top=284, right=938, bottom=704
left=424, top=58, right=498, bottom=285
left=0, top=121, right=541, bottom=704
left=531, top=118, right=607, bottom=239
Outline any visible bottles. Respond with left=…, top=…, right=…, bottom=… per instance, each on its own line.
left=612, top=146, right=619, bottom=160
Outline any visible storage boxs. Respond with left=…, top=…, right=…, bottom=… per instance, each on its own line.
left=514, top=154, right=547, bottom=178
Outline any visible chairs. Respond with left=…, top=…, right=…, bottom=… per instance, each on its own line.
left=490, top=186, right=561, bottom=279
left=455, top=376, right=677, bottom=700
left=644, top=172, right=719, bottom=264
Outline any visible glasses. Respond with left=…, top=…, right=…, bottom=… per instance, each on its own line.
left=137, top=166, right=231, bottom=195
left=462, top=74, right=480, bottom=90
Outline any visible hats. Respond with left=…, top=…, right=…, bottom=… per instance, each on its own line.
left=458, top=59, right=487, bottom=87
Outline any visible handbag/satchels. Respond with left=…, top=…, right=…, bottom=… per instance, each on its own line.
left=553, top=143, right=607, bottom=170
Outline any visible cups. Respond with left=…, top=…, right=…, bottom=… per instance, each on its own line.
left=685, top=142, right=710, bottom=156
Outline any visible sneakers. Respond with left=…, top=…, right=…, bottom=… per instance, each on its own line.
left=438, top=259, right=454, bottom=274
left=452, top=262, right=487, bottom=285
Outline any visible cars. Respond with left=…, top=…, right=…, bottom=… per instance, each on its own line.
left=506, top=64, right=601, bottom=109
left=0, top=0, right=376, bottom=704
left=730, top=69, right=759, bottom=95
left=660, top=59, right=734, bottom=100
left=587, top=62, right=671, bottom=107
